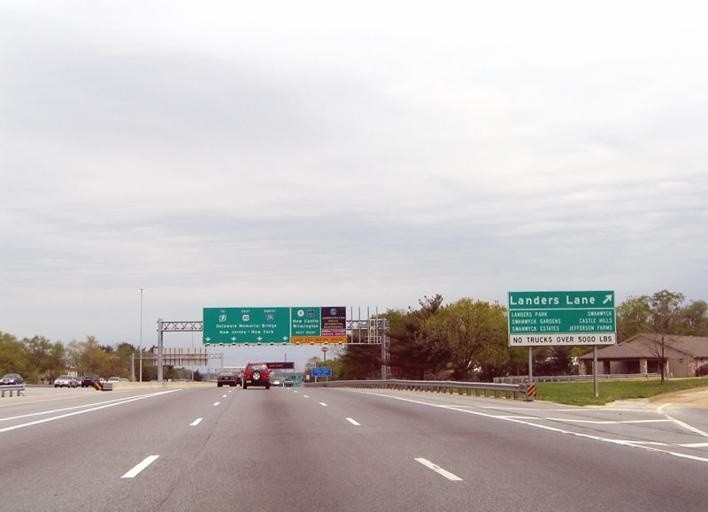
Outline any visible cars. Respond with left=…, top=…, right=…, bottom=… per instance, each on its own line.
left=53, top=374, right=130, bottom=388
left=0, top=372, right=24, bottom=385
left=216, top=371, right=242, bottom=387
left=272, top=379, right=293, bottom=387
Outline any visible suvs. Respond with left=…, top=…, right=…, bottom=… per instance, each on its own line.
left=241, top=362, right=272, bottom=389
